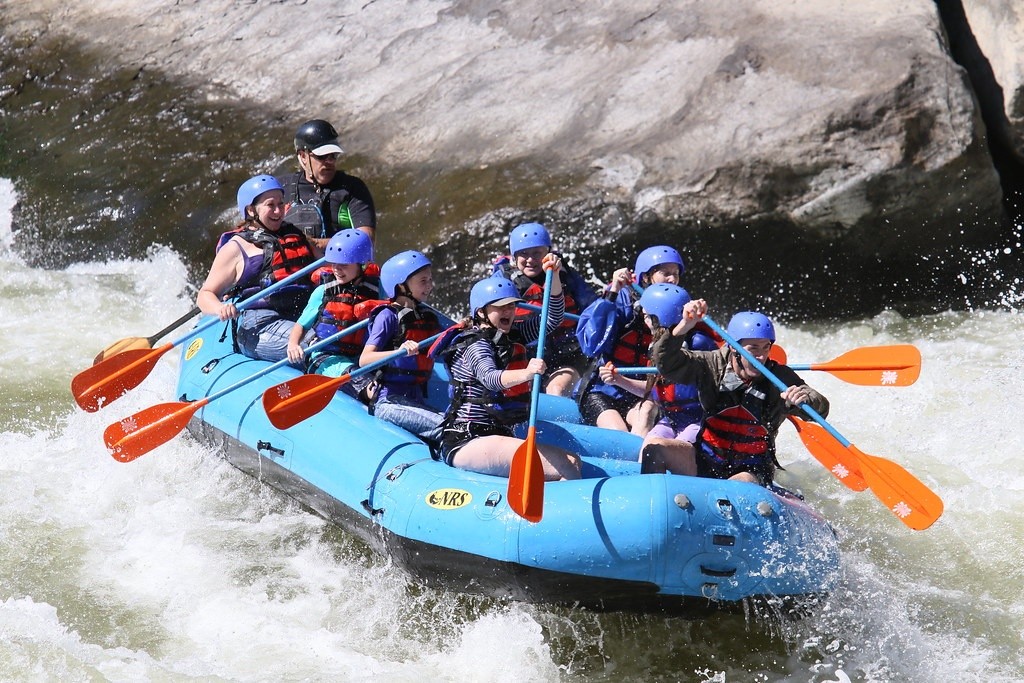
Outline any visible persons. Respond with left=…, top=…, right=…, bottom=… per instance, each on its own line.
left=280, top=120, right=376, bottom=252
left=197, top=176, right=828, bottom=490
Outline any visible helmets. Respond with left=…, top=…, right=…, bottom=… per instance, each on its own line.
left=638, top=283, right=692, bottom=327
left=726, top=311, right=776, bottom=350
left=294, top=119, right=343, bottom=147
left=470, top=276, right=521, bottom=319
left=324, top=228, right=376, bottom=266
left=633, top=245, right=685, bottom=287
left=237, top=176, right=285, bottom=221
left=378, top=249, right=432, bottom=299
left=509, top=223, right=552, bottom=261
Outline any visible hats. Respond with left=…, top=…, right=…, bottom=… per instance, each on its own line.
left=310, top=144, right=344, bottom=156
left=490, top=296, right=527, bottom=306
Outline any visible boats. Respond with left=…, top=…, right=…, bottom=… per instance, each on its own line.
left=177, top=297, right=841, bottom=616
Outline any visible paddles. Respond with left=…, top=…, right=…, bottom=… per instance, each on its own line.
left=262, top=330, right=444, bottom=430
left=626, top=274, right=787, bottom=364
left=103, top=318, right=370, bottom=464
left=788, top=416, right=871, bottom=493
left=507, top=264, right=553, bottom=522
left=93, top=305, right=202, bottom=364
left=516, top=301, right=581, bottom=320
left=70, top=256, right=327, bottom=414
left=606, top=345, right=921, bottom=387
left=688, top=309, right=944, bottom=531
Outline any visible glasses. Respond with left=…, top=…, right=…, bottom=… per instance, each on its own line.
left=309, top=151, right=337, bottom=164
